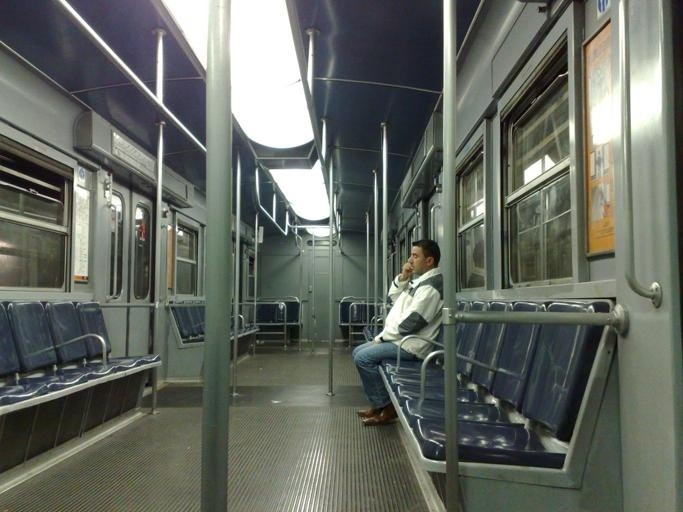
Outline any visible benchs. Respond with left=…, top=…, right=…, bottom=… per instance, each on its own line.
left=0, top=295, right=163, bottom=464
left=338, top=288, right=615, bottom=490
left=164, top=296, right=301, bottom=383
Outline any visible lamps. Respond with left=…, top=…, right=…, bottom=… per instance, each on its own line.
left=151, top=2, right=317, bottom=150
left=266, top=150, right=339, bottom=238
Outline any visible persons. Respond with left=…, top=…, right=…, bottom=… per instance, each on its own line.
left=354, top=237, right=445, bottom=424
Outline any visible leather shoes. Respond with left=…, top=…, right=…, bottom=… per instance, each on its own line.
left=357, top=407, right=376, bottom=418
left=362, top=405, right=399, bottom=426
